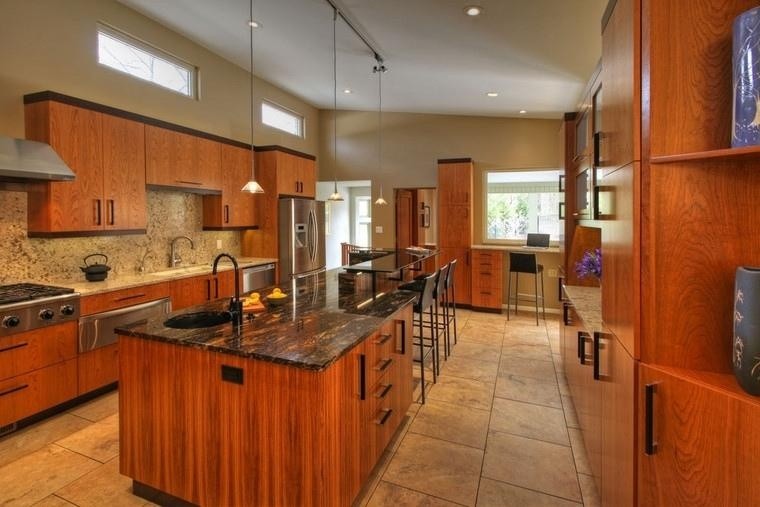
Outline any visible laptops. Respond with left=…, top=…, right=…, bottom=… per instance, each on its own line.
left=522, top=233, right=550, bottom=249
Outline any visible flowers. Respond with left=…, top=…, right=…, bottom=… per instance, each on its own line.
left=573, top=247, right=601, bottom=279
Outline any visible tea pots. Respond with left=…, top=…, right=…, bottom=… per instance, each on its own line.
left=78, top=253, right=112, bottom=282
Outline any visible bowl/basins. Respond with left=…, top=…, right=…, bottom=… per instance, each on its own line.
left=267, top=294, right=288, bottom=306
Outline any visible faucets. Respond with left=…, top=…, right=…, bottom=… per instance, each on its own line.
left=211, top=253, right=246, bottom=328
left=168, top=235, right=194, bottom=268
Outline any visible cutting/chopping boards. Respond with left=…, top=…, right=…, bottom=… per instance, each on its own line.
left=230, top=296, right=265, bottom=312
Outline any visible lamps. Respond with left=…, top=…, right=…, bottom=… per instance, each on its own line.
left=243, top=0, right=265, bottom=195
left=326, top=8, right=345, bottom=202
left=372, top=55, right=388, bottom=205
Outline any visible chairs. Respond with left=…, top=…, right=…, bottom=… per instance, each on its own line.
left=398, top=259, right=456, bottom=403
left=507, top=252, right=545, bottom=326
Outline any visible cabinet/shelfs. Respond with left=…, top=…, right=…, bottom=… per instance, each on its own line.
left=202, top=138, right=260, bottom=230
left=393, top=157, right=504, bottom=314
left=144, top=118, right=224, bottom=195
left=243, top=146, right=316, bottom=257
left=115, top=298, right=415, bottom=507
left=0, top=320, right=79, bottom=431
left=557, top=0, right=760, bottom=507
left=23, top=90, right=147, bottom=238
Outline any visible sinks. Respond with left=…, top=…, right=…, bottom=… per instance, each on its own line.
left=164, top=309, right=233, bottom=330
left=151, top=265, right=213, bottom=278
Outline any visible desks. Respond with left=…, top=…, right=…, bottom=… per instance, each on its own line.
left=343, top=247, right=443, bottom=301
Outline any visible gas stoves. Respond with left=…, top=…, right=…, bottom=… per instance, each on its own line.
left=0, top=282, right=80, bottom=337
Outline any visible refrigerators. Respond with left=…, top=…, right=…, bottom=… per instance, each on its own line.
left=279, top=197, right=326, bottom=285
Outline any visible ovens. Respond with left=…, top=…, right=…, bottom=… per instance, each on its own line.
left=244, top=262, right=275, bottom=295
